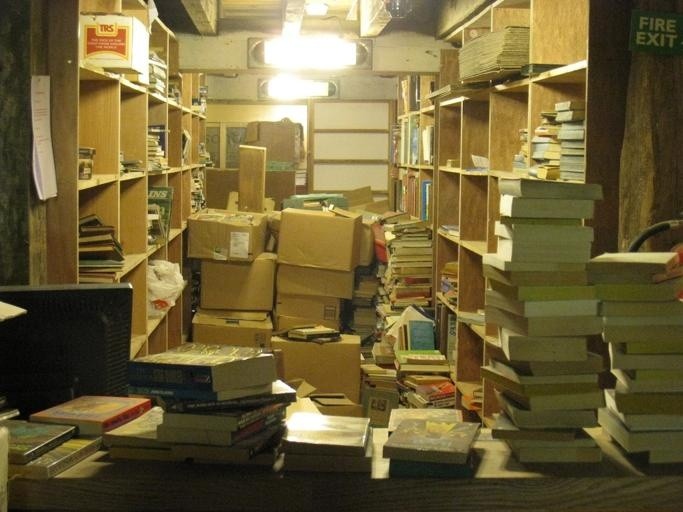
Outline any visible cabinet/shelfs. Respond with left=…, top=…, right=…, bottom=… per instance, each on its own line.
left=393, top=72, right=435, bottom=244
left=435, top=1, right=587, bottom=428
left=162, top=23, right=183, bottom=359
left=47, top=0, right=161, bottom=358
left=183, top=71, right=209, bottom=350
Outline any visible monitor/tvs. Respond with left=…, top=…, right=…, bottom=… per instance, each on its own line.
left=0, top=283, right=133, bottom=423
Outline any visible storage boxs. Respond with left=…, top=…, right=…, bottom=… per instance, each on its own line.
left=187, top=185, right=281, bottom=354
left=270, top=207, right=363, bottom=407
left=283, top=185, right=392, bottom=277
left=76, top=9, right=148, bottom=76
